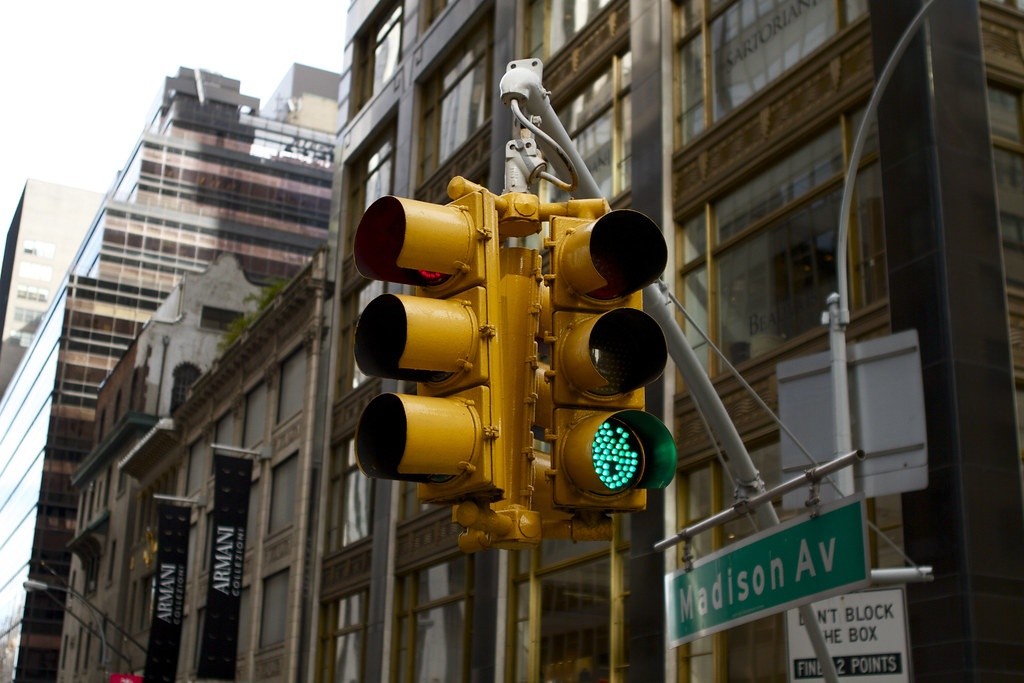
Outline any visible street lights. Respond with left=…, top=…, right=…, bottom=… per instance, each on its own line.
left=21, top=579, right=105, bottom=683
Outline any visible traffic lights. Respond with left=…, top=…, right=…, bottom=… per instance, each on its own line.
left=545, top=208, right=681, bottom=511
left=351, top=185, right=522, bottom=504
